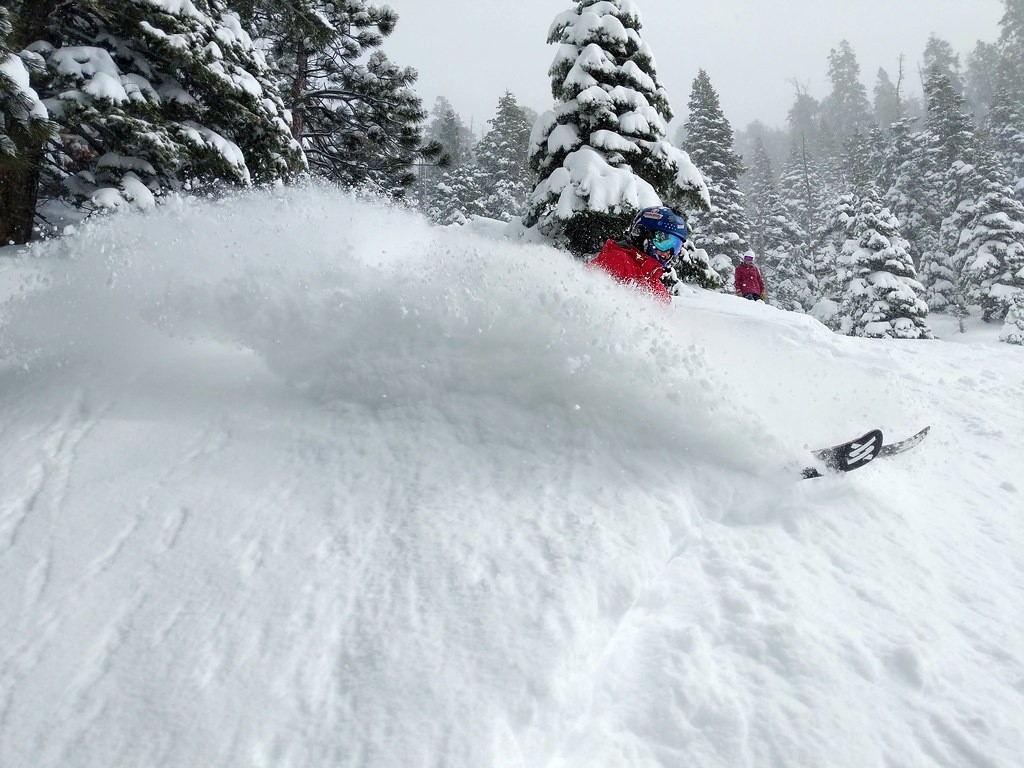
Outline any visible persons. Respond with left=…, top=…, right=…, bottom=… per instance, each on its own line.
left=583, top=204, right=687, bottom=309
left=734, top=249, right=766, bottom=304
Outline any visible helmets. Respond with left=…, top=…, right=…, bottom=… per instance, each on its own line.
left=743, top=250, right=755, bottom=260
left=622, top=205, right=687, bottom=267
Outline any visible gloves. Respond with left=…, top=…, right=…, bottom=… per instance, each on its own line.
left=760, top=292, right=767, bottom=300
left=737, top=291, right=743, bottom=297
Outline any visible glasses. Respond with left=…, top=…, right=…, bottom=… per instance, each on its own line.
left=652, top=231, right=683, bottom=257
left=745, top=256, right=753, bottom=262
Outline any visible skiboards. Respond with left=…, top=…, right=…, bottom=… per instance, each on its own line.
left=796, top=425, right=928, bottom=487
